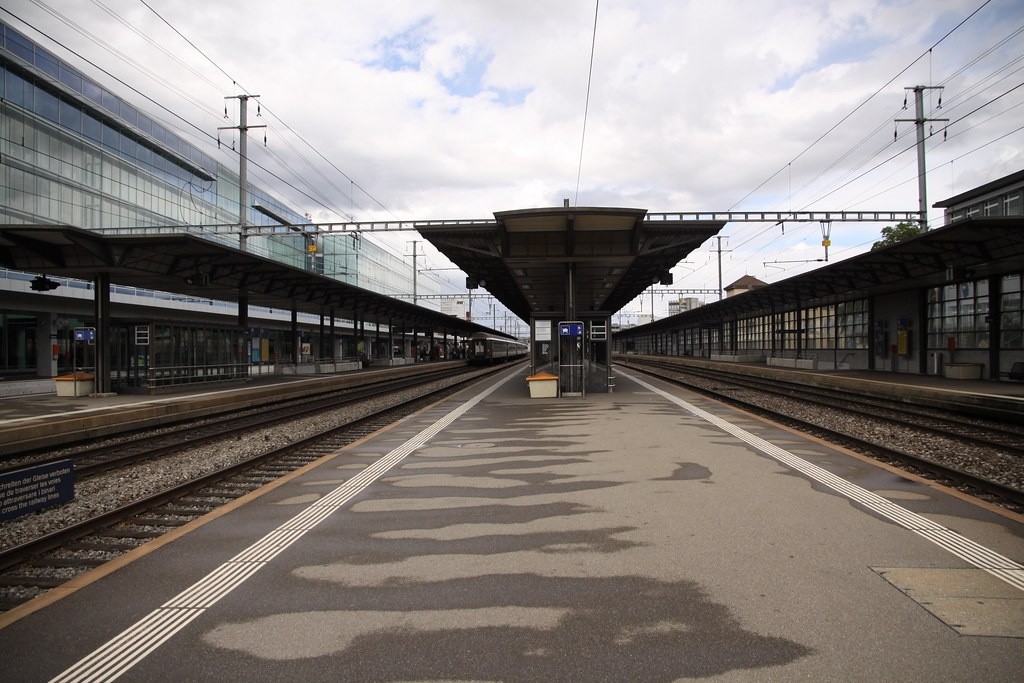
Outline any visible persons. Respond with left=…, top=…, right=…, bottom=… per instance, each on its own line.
left=419, top=347, right=426, bottom=361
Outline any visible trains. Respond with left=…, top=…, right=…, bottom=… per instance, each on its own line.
left=466, top=332, right=529, bottom=365
